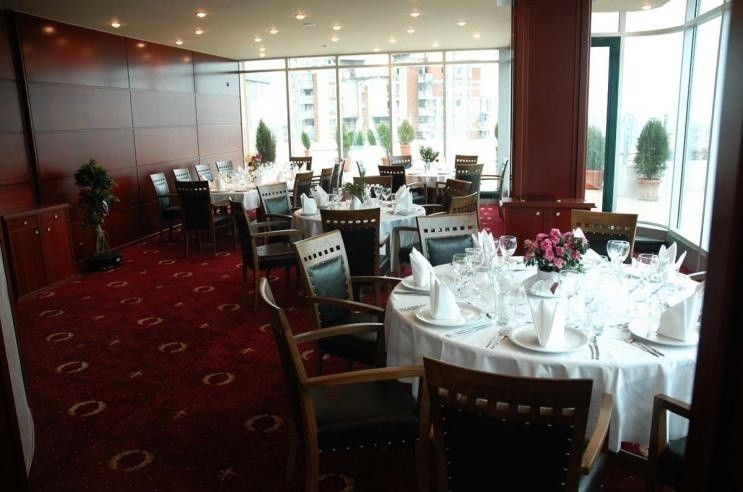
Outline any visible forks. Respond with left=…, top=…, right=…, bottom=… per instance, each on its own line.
left=588, top=335, right=599, bottom=361
left=485, top=328, right=508, bottom=349
left=623, top=330, right=663, bottom=357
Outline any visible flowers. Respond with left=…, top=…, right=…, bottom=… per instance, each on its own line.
left=523, top=228, right=590, bottom=273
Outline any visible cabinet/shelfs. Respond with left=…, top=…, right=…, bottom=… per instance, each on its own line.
left=503, top=198, right=595, bottom=257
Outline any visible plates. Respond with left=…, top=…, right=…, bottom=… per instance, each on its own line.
left=508, top=322, right=588, bottom=353
left=209, top=188, right=228, bottom=192
left=298, top=210, right=319, bottom=216
left=401, top=274, right=454, bottom=295
left=414, top=303, right=484, bottom=327
left=627, top=312, right=700, bottom=348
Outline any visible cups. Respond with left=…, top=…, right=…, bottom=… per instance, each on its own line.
left=606, top=240, right=630, bottom=263
left=493, top=237, right=499, bottom=252
left=452, top=253, right=469, bottom=299
left=637, top=253, right=659, bottom=280
left=380, top=187, right=392, bottom=206
left=498, top=235, right=517, bottom=263
left=465, top=248, right=483, bottom=280
left=373, top=186, right=382, bottom=200
left=333, top=187, right=342, bottom=204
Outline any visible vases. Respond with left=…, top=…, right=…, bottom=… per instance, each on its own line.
left=535, top=267, right=558, bottom=284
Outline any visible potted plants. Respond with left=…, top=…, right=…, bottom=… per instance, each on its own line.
left=334, top=123, right=354, bottom=172
left=397, top=119, right=414, bottom=157
left=259, top=278, right=430, bottom=492
left=376, top=124, right=394, bottom=166
left=419, top=145, right=440, bottom=172
left=633, top=118, right=669, bottom=201
left=301, top=132, right=310, bottom=156
left=71, top=157, right=122, bottom=271
left=585, top=126, right=603, bottom=192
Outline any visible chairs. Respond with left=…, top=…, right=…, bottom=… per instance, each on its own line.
left=215, top=161, right=234, bottom=178
left=292, top=230, right=402, bottom=376
left=354, top=176, right=393, bottom=198
left=383, top=155, right=412, bottom=169
left=320, top=207, right=391, bottom=309
left=293, top=173, right=313, bottom=210
left=338, top=160, right=345, bottom=189
left=320, top=168, right=333, bottom=201
left=194, top=165, right=214, bottom=182
left=149, top=171, right=184, bottom=242
left=570, top=209, right=639, bottom=264
left=174, top=181, right=237, bottom=260
left=229, top=198, right=302, bottom=313
left=379, top=165, right=427, bottom=212
left=333, top=162, right=339, bottom=189
left=172, top=168, right=192, bottom=182
left=289, top=156, right=312, bottom=170
left=423, top=355, right=612, bottom=492
left=449, top=192, right=478, bottom=217
left=436, top=165, right=484, bottom=231
left=339, top=159, right=347, bottom=189
left=256, top=182, right=300, bottom=245
left=355, top=160, right=367, bottom=177
left=615, top=390, right=696, bottom=492
left=415, top=212, right=479, bottom=266
left=455, top=155, right=478, bottom=164
